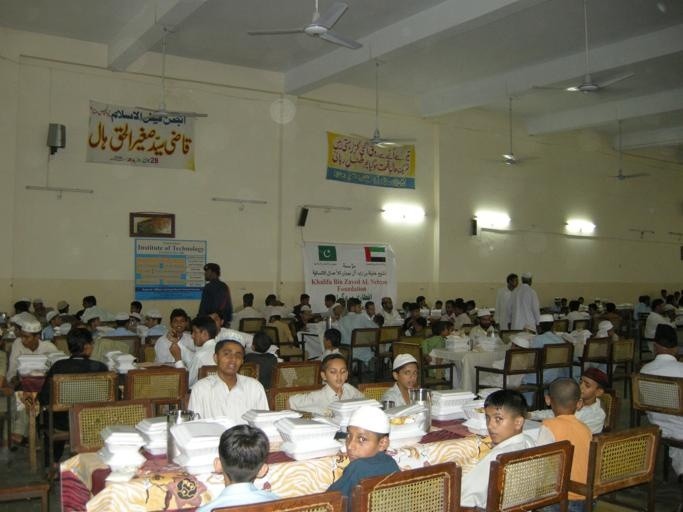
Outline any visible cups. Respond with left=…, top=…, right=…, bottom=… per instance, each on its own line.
left=382, top=401, right=395, bottom=411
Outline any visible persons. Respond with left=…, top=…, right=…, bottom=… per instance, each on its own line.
left=326, top=404, right=402, bottom=512
left=541, top=376, right=599, bottom=511
left=379, top=353, right=419, bottom=411
left=196, top=265, right=233, bottom=329
left=548, top=296, right=623, bottom=333
left=196, top=425, right=282, bottom=512
left=461, top=389, right=537, bottom=511
left=525, top=366, right=608, bottom=435
left=235, top=292, right=265, bottom=329
left=34, top=327, right=108, bottom=482
left=285, top=354, right=366, bottom=417
left=187, top=340, right=270, bottom=417
left=154, top=308, right=284, bottom=394
left=507, top=271, right=541, bottom=335
left=493, top=273, right=519, bottom=331
left=638, top=324, right=683, bottom=477
left=3, top=296, right=168, bottom=450
left=632, top=288, right=680, bottom=351
left=262, top=294, right=496, bottom=384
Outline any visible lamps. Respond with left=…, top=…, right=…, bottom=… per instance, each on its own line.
left=46, top=122, right=66, bottom=155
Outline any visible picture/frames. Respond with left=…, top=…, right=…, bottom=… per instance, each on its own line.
left=129, top=211, right=175, bottom=238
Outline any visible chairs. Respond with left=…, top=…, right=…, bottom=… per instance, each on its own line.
left=0, top=308, right=683, bottom=512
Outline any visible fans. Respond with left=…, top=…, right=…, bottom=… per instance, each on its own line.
left=133, top=25, right=208, bottom=123
left=247, top=0, right=363, bottom=50
left=603, top=120, right=652, bottom=181
left=349, top=58, right=419, bottom=149
left=478, top=96, right=543, bottom=167
left=530, top=0, right=640, bottom=96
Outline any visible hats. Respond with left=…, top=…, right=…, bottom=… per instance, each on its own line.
left=147, top=310, right=161, bottom=318
left=654, top=323, right=677, bottom=348
left=598, top=320, right=613, bottom=330
left=46, top=310, right=59, bottom=322
left=114, top=312, right=128, bottom=321
left=129, top=312, right=140, bottom=320
left=57, top=300, right=68, bottom=310
left=521, top=272, right=531, bottom=278
left=22, top=319, right=41, bottom=333
left=15, top=311, right=36, bottom=326
left=582, top=367, right=609, bottom=385
left=392, top=352, right=418, bottom=370
left=554, top=297, right=560, bottom=303
left=477, top=309, right=489, bottom=317
left=215, top=331, right=245, bottom=347
left=346, top=404, right=390, bottom=434
